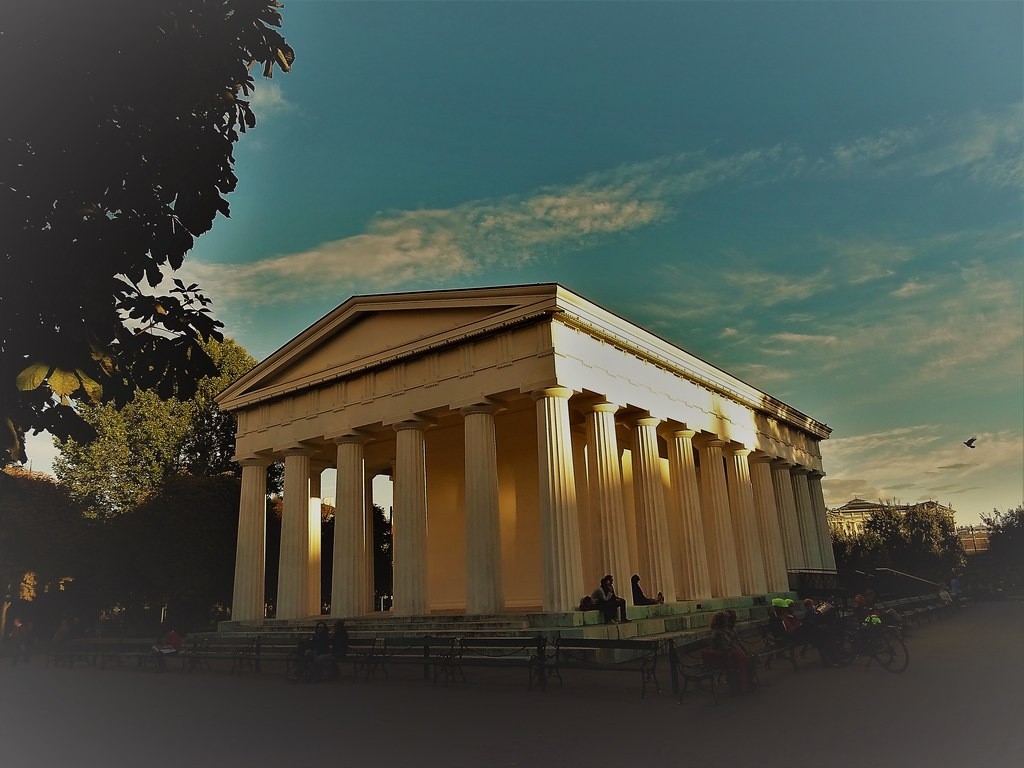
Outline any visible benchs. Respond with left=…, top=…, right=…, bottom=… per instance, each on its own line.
left=669, top=589, right=976, bottom=691
left=68, top=629, right=662, bottom=701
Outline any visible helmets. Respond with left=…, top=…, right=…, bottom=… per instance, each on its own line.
left=771, top=598, right=789, bottom=607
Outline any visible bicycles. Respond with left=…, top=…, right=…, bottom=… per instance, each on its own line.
left=811, top=591, right=911, bottom=675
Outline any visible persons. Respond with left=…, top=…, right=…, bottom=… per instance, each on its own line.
left=630, top=573, right=664, bottom=605
left=303, top=619, right=348, bottom=683
left=770, top=598, right=850, bottom=666
left=285, top=622, right=330, bottom=681
left=158, top=626, right=183, bottom=669
left=39, top=614, right=104, bottom=669
left=605, top=574, right=632, bottom=623
left=950, top=575, right=961, bottom=590
left=710, top=609, right=750, bottom=676
left=590, top=577, right=618, bottom=626
left=861, top=590, right=913, bottom=638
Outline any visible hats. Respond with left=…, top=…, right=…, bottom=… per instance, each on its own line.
left=786, top=599, right=794, bottom=605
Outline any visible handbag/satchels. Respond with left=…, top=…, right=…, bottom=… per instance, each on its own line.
left=580, top=595, right=591, bottom=610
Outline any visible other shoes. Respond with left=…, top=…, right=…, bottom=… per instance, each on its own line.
left=658, top=592, right=663, bottom=603
left=608, top=618, right=622, bottom=625
left=622, top=619, right=632, bottom=623
left=661, top=596, right=664, bottom=604
